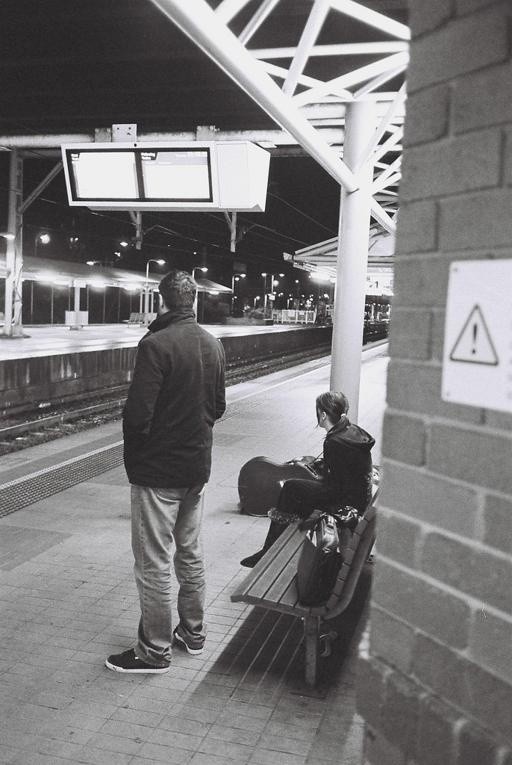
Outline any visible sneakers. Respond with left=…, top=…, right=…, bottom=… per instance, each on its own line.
left=173, top=627, right=205, bottom=655
left=105, top=648, right=170, bottom=674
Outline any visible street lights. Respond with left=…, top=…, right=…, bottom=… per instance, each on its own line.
left=286, top=292, right=330, bottom=311
left=260, top=271, right=283, bottom=312
left=230, top=273, right=247, bottom=316
left=366, top=299, right=391, bottom=321
left=27, top=230, right=50, bottom=321
left=141, top=258, right=168, bottom=323
left=190, top=267, right=211, bottom=325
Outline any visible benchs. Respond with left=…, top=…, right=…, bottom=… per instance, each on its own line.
left=230, top=464, right=380, bottom=699
left=123, top=312, right=157, bottom=327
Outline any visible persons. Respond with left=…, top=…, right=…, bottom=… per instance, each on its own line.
left=106, top=271, right=229, bottom=674
left=241, top=391, right=375, bottom=569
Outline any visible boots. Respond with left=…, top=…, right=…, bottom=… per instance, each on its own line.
left=240, top=509, right=298, bottom=568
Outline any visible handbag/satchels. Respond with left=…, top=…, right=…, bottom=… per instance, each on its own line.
left=297, top=510, right=343, bottom=608
